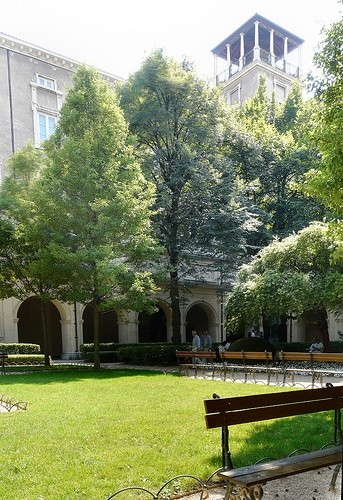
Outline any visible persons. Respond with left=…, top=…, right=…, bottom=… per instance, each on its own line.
left=202, top=331, right=212, bottom=363
left=192, top=331, right=203, bottom=369
left=308, top=337, right=324, bottom=353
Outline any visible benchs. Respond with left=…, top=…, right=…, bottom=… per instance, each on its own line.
left=204, top=383, right=343, bottom=500
left=279, top=352, right=343, bottom=388
left=218, top=350, right=281, bottom=384
left=177, top=351, right=222, bottom=380
